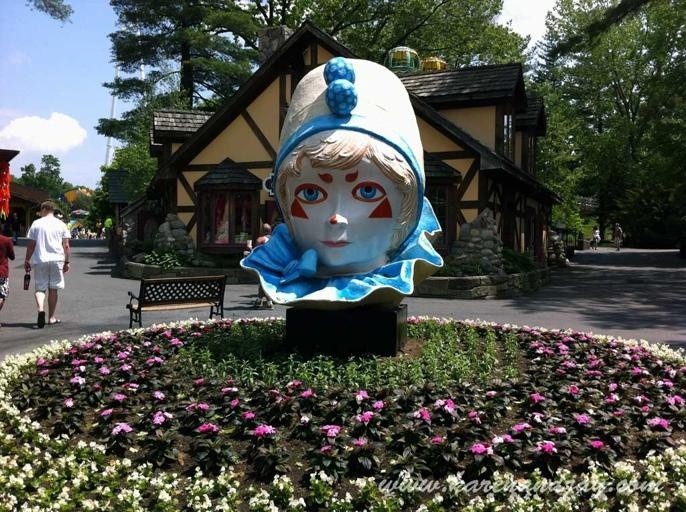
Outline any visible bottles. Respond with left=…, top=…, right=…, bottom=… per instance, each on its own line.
left=22, top=268, right=30, bottom=292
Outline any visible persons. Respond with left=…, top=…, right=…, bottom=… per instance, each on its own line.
left=613, top=222, right=622, bottom=251
left=71, top=216, right=132, bottom=240
left=23, top=201, right=71, bottom=328
left=0, top=235, right=15, bottom=328
left=252, top=223, right=273, bottom=308
left=239, top=60, right=445, bottom=310
left=591, top=226, right=600, bottom=251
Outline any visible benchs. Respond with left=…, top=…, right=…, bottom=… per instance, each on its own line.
left=123, top=272, right=228, bottom=330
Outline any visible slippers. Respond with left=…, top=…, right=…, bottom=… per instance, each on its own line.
left=49, top=318, right=61, bottom=325
left=37, top=310, right=46, bottom=329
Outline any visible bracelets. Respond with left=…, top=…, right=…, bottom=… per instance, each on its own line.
left=65, top=262, right=69, bottom=264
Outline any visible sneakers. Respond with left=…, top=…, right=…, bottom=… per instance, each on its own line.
left=254, top=298, right=275, bottom=310
